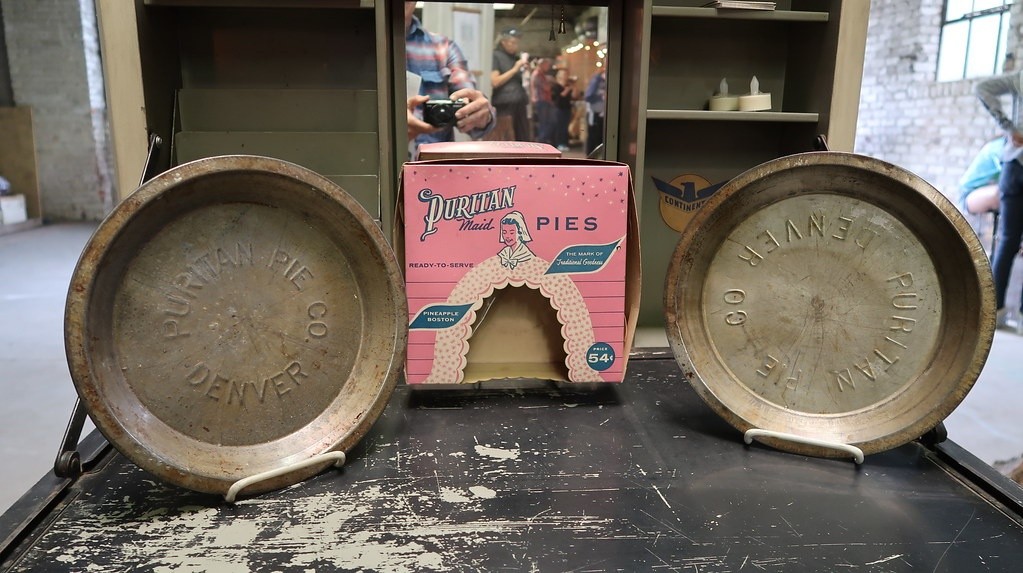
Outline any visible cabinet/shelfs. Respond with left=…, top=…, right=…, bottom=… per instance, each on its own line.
left=618, top=0, right=873, bottom=349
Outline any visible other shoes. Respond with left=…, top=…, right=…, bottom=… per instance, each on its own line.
left=995, top=313, right=1008, bottom=328
left=558, top=145, right=570, bottom=152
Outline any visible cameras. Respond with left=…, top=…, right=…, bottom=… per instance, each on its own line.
left=423, top=97, right=469, bottom=127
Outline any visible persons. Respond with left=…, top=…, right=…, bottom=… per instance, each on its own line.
left=405, top=1, right=496, bottom=164
left=958, top=69, right=1023, bottom=335
left=491, top=25, right=605, bottom=158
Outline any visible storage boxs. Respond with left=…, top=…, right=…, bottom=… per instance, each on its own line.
left=393, top=157, right=643, bottom=386
left=416, top=141, right=562, bottom=159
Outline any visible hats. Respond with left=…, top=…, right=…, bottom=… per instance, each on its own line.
left=504, top=25, right=522, bottom=37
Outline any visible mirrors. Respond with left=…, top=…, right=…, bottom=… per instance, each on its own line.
left=391, top=0, right=622, bottom=162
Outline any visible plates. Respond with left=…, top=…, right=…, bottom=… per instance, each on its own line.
left=663, top=151, right=998, bottom=464
left=64, top=155, right=409, bottom=497
